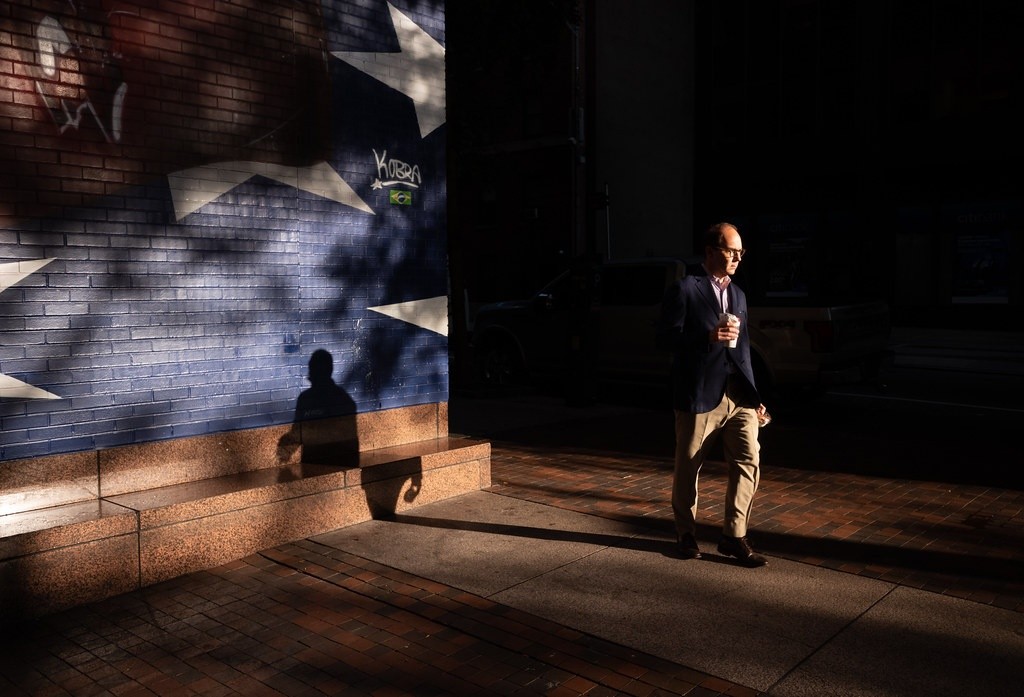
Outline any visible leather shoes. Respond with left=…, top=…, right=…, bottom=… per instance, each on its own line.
left=717, top=534, right=770, bottom=567
left=678, top=532, right=702, bottom=559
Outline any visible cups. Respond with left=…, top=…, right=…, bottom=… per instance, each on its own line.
left=720, top=314, right=740, bottom=348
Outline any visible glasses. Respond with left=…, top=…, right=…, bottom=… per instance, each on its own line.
left=714, top=248, right=745, bottom=257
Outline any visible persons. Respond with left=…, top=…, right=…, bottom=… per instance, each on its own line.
left=671, top=222, right=773, bottom=567
